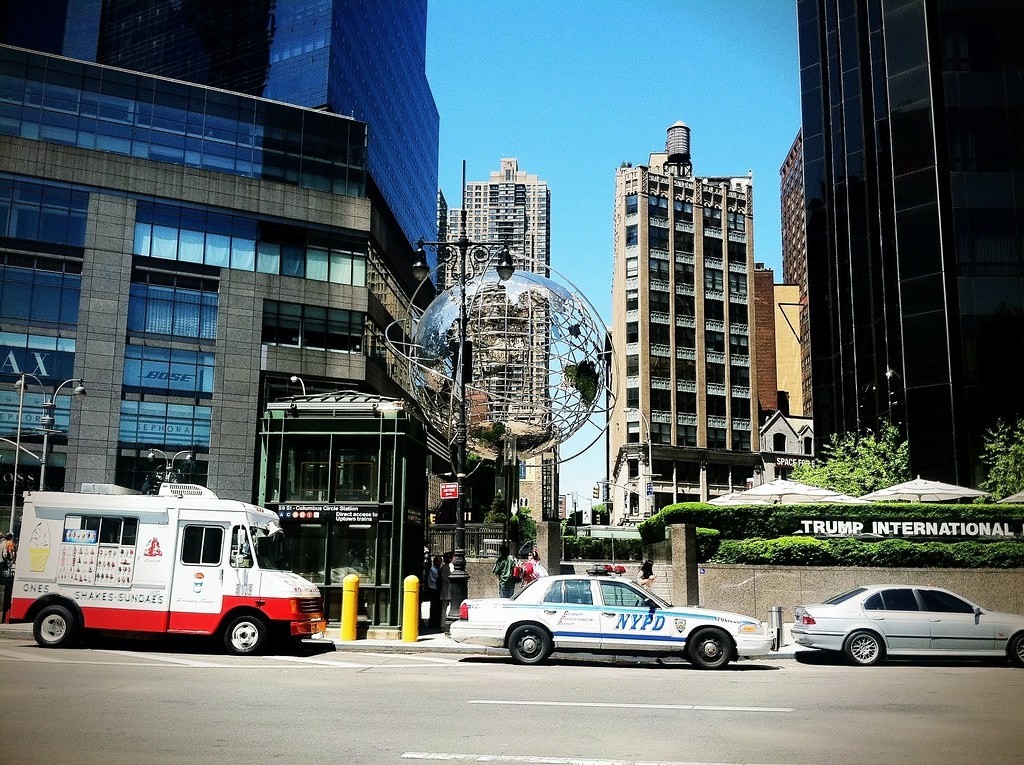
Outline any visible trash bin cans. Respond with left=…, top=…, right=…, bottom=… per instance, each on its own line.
left=766, top=605, right=785, bottom=647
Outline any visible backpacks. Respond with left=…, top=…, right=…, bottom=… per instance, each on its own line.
left=526, top=559, right=549, bottom=579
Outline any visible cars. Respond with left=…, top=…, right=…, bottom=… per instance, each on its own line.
left=448, top=561, right=776, bottom=669
left=790, top=583, right=1024, bottom=667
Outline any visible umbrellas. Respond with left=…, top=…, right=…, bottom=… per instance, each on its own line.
left=997, top=488, right=1024, bottom=504
left=703, top=476, right=875, bottom=507
left=856, top=475, right=993, bottom=504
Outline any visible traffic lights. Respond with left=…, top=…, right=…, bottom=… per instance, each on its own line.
left=592, top=486, right=601, bottom=499
left=595, top=514, right=601, bottom=526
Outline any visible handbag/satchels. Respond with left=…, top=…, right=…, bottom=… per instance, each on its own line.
left=5, top=552, right=13, bottom=561
left=513, top=559, right=523, bottom=579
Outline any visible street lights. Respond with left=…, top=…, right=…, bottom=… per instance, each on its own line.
left=0, top=370, right=87, bottom=488
left=414, top=159, right=516, bottom=634
left=624, top=406, right=654, bottom=513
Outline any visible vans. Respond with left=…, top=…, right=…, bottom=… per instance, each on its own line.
left=8, top=481, right=328, bottom=653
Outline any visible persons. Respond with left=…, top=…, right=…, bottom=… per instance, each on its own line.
left=438, top=552, right=453, bottom=632
left=491, top=545, right=519, bottom=598
left=427, top=556, right=442, bottom=633
left=5, top=533, right=15, bottom=568
left=636, top=559, right=657, bottom=592
left=0, top=532, right=7, bottom=572
left=517, top=551, right=541, bottom=589
